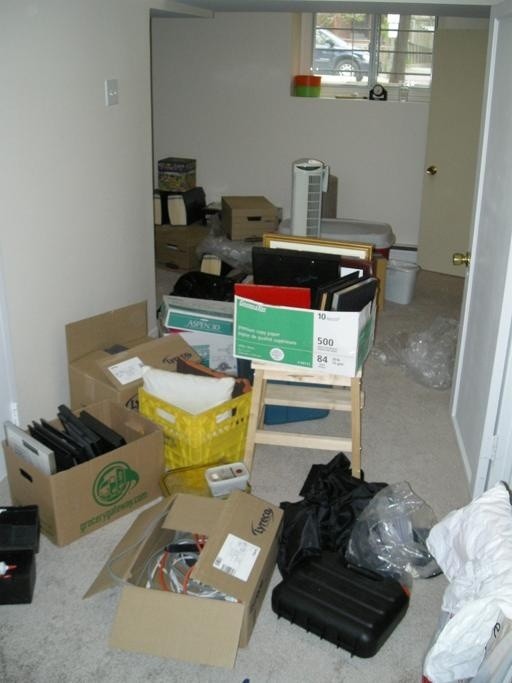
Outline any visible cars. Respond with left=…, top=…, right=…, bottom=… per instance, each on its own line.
left=313, top=26, right=376, bottom=80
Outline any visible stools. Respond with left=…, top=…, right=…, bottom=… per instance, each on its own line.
left=243, top=361, right=364, bottom=481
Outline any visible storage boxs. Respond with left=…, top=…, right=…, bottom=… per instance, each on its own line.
left=233, top=289, right=377, bottom=377
left=237, top=359, right=331, bottom=425
left=157, top=315, right=237, bottom=376
left=158, top=158, right=195, bottom=191
left=281, top=218, right=395, bottom=259
left=66, top=300, right=204, bottom=404
left=155, top=225, right=209, bottom=270
left=222, top=196, right=278, bottom=240
left=0, top=400, right=164, bottom=548
left=84, top=488, right=284, bottom=668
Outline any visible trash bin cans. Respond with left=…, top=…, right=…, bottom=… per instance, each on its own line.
left=384, top=259, right=421, bottom=305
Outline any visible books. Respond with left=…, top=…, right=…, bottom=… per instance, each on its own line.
left=314, top=271, right=380, bottom=311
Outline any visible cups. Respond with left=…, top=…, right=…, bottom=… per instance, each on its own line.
left=399, top=86, right=409, bottom=101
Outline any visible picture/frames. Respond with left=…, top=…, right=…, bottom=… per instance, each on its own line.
left=264, top=233, right=374, bottom=263
